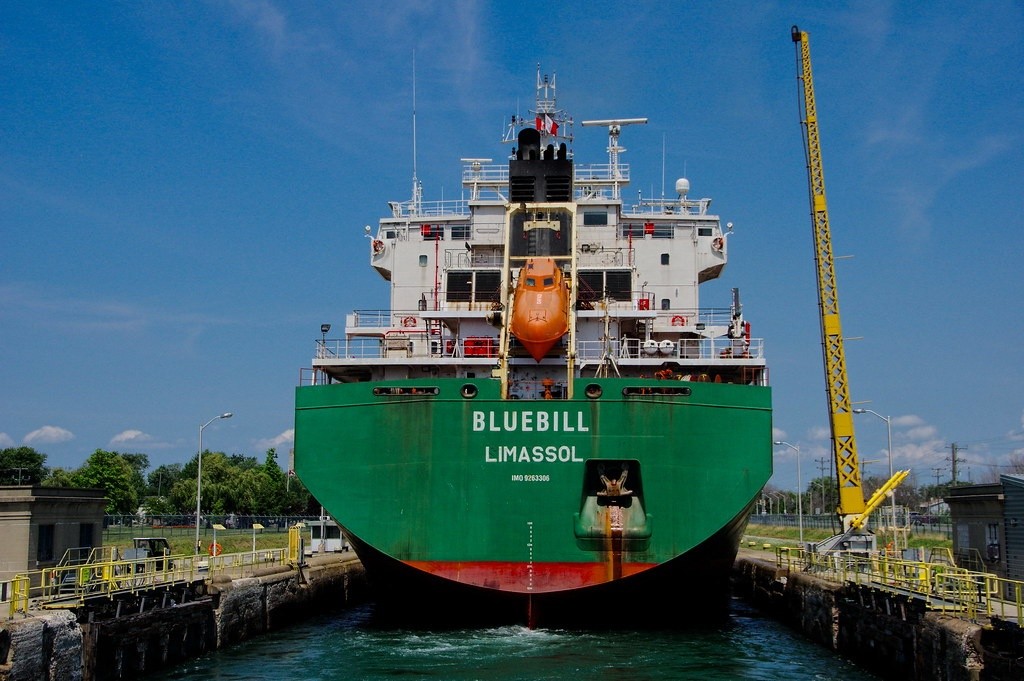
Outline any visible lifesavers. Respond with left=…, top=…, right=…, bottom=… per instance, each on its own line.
left=672, top=315, right=684, bottom=326
left=404, top=316, right=416, bottom=327
left=373, top=240, right=385, bottom=252
left=887, top=541, right=896, bottom=557
left=208, top=543, right=222, bottom=556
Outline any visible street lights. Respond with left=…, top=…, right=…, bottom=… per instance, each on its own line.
left=194, top=412, right=233, bottom=558
left=852, top=408, right=897, bottom=540
left=776, top=440, right=805, bottom=545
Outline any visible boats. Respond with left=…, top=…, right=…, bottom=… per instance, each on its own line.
left=293, top=61, right=771, bottom=624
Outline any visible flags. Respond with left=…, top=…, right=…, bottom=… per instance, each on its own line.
left=546, top=115, right=559, bottom=136
left=536, top=116, right=546, bottom=131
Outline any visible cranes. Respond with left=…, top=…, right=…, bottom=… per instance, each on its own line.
left=791, top=21, right=878, bottom=566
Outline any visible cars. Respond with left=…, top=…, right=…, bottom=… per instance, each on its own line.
left=115, top=536, right=175, bottom=572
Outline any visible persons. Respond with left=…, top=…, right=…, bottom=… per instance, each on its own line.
left=197, top=539, right=202, bottom=554
left=139, top=542, right=149, bottom=551
left=886, top=540, right=895, bottom=557
